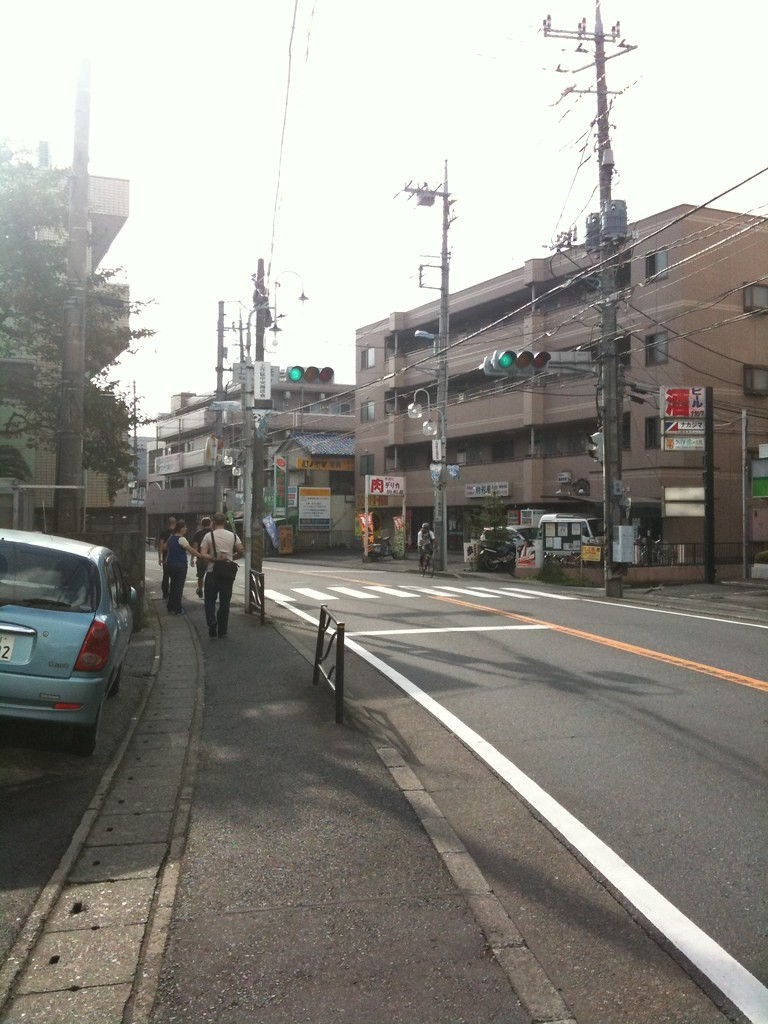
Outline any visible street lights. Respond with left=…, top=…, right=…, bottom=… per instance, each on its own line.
left=243, top=269, right=309, bottom=614
left=406, top=388, right=449, bottom=571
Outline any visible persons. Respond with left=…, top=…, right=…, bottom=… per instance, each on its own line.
left=157, top=517, right=213, bottom=614
left=200, top=512, right=244, bottom=639
left=418, top=523, right=437, bottom=569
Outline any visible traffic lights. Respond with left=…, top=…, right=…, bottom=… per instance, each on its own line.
left=497, top=348, right=552, bottom=372
left=587, top=431, right=604, bottom=463
left=285, top=365, right=334, bottom=384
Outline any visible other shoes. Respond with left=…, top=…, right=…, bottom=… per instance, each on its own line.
left=218, top=631, right=227, bottom=638
left=167, top=604, right=174, bottom=611
left=209, top=620, right=217, bottom=637
left=163, top=593, right=168, bottom=598
left=175, top=607, right=180, bottom=614
left=196, top=589, right=203, bottom=598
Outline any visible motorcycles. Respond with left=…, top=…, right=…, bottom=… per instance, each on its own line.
left=479, top=544, right=518, bottom=570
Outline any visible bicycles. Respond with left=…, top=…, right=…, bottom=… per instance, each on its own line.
left=417, top=545, right=438, bottom=578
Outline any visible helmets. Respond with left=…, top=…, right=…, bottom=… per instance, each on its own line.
left=422, top=523, right=430, bottom=530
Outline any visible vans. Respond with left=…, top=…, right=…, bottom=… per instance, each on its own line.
left=483, top=525, right=538, bottom=557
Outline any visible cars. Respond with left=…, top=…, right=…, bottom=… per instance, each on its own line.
left=0, top=528, right=140, bottom=756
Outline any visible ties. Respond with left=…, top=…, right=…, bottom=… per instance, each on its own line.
left=524, top=547, right=526, bottom=557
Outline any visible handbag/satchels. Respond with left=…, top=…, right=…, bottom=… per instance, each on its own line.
left=163, top=565, right=175, bottom=577
left=215, top=560, right=240, bottom=580
